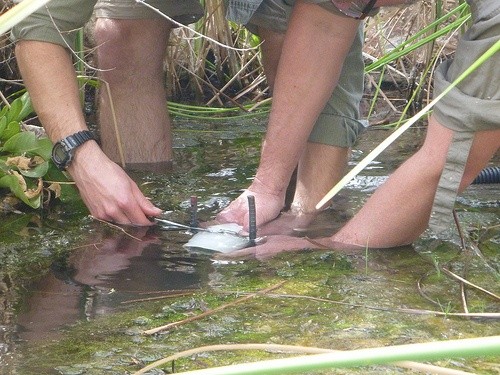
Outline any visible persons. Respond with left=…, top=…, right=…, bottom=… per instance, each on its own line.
left=11, top=1, right=368, bottom=243
left=202, top=0, right=499, bottom=263
left=13, top=220, right=164, bottom=356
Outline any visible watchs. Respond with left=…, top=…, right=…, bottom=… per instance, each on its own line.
left=52, top=128, right=98, bottom=170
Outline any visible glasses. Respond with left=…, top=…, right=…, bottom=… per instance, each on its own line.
left=329, top=0, right=377, bottom=20
